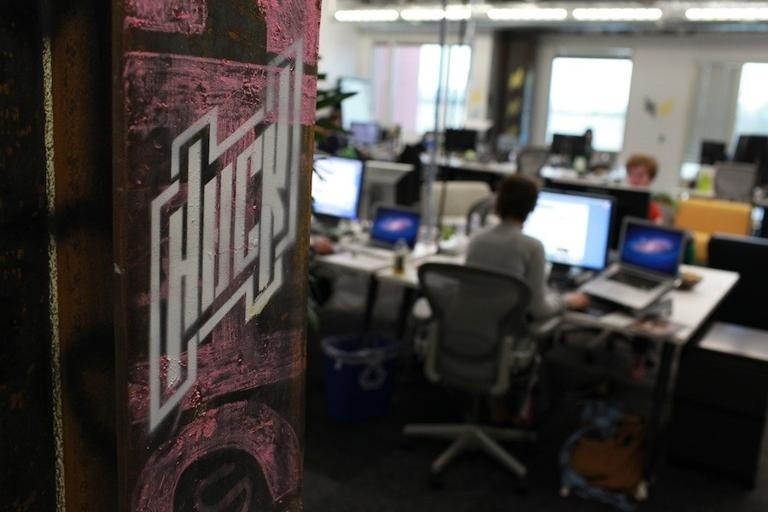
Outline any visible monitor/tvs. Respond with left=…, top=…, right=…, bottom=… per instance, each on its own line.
left=310, top=152, right=366, bottom=241
left=520, top=187, right=615, bottom=290
left=349, top=121, right=381, bottom=147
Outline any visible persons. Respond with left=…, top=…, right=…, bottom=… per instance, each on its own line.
left=463, top=174, right=589, bottom=423
left=625, top=154, right=664, bottom=226
left=584, top=129, right=595, bottom=157
left=396, top=142, right=429, bottom=207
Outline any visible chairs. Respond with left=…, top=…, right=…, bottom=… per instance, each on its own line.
left=402, top=257, right=563, bottom=479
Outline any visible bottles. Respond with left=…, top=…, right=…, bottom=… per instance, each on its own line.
left=393, top=238, right=409, bottom=274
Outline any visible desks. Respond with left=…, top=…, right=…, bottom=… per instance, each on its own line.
left=312, top=217, right=449, bottom=372
left=376, top=243, right=739, bottom=479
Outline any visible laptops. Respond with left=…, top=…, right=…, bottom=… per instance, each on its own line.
left=577, top=216, right=688, bottom=311
left=343, top=206, right=422, bottom=261
